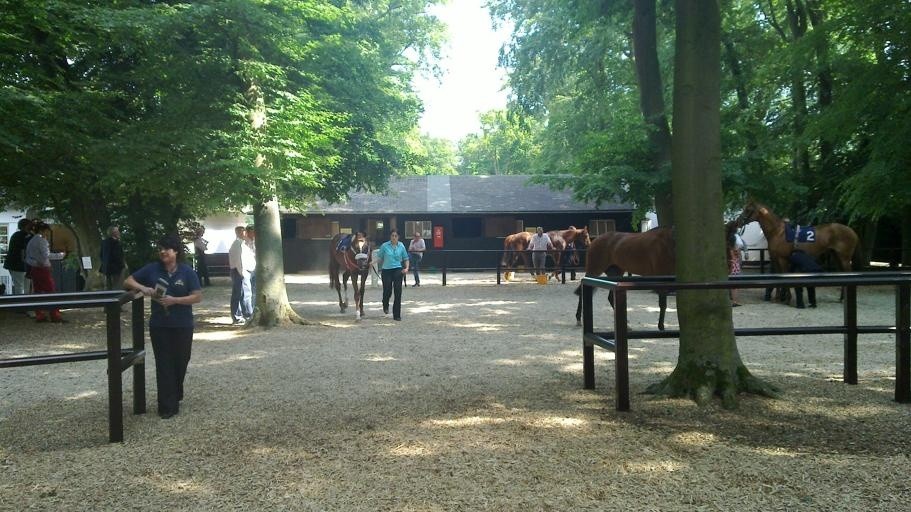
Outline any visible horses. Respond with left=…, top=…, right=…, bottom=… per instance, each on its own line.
left=502, top=225, right=591, bottom=282
left=575, top=225, right=677, bottom=331
left=735, top=195, right=865, bottom=303
left=329, top=230, right=372, bottom=322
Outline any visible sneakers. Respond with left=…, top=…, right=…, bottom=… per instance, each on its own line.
left=394, top=316, right=401, bottom=321
left=383, top=306, right=389, bottom=314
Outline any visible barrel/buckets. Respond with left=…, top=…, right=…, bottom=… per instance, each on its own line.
left=504, top=272, right=515, bottom=281
left=535, top=274, right=547, bottom=284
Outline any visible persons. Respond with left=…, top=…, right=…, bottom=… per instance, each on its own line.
left=407, top=231, right=427, bottom=287
left=525, top=226, right=557, bottom=275
left=788, top=249, right=826, bottom=309
left=4, top=217, right=258, bottom=325
left=368, top=228, right=410, bottom=321
left=122, top=232, right=205, bottom=418
left=725, top=220, right=744, bottom=308
left=558, top=225, right=578, bottom=264
